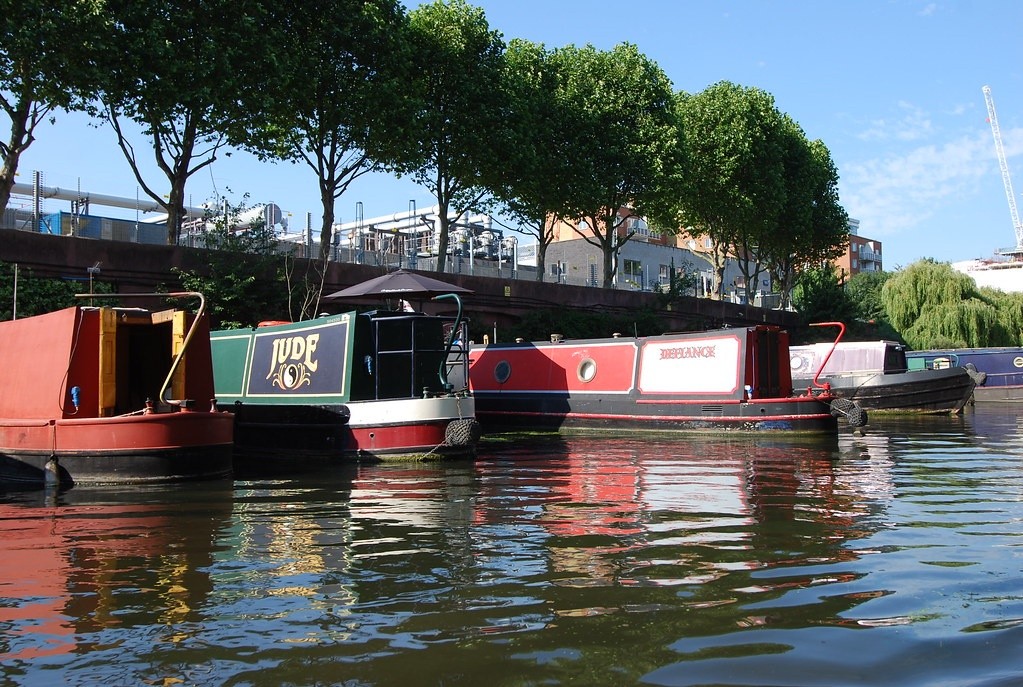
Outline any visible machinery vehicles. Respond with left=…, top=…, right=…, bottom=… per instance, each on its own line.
left=982, top=83, right=1023, bottom=270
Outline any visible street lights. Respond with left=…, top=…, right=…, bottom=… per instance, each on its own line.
left=87, top=262, right=102, bottom=307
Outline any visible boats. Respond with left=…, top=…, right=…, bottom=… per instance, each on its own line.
left=209, top=269, right=481, bottom=454
left=469, top=314, right=867, bottom=435
left=787, top=341, right=1023, bottom=416
left=0, top=292, right=235, bottom=488
